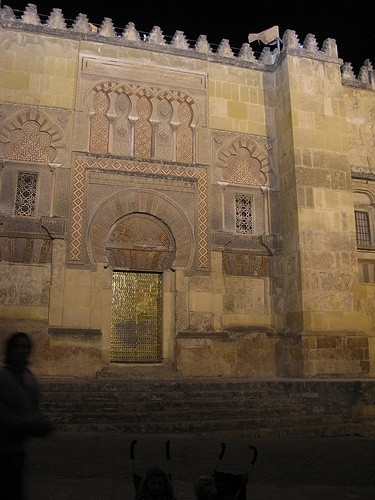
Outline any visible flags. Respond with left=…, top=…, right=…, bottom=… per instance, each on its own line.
left=248, top=26, right=278, bottom=48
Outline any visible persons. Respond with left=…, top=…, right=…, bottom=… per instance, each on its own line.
left=0, top=329, right=46, bottom=500
